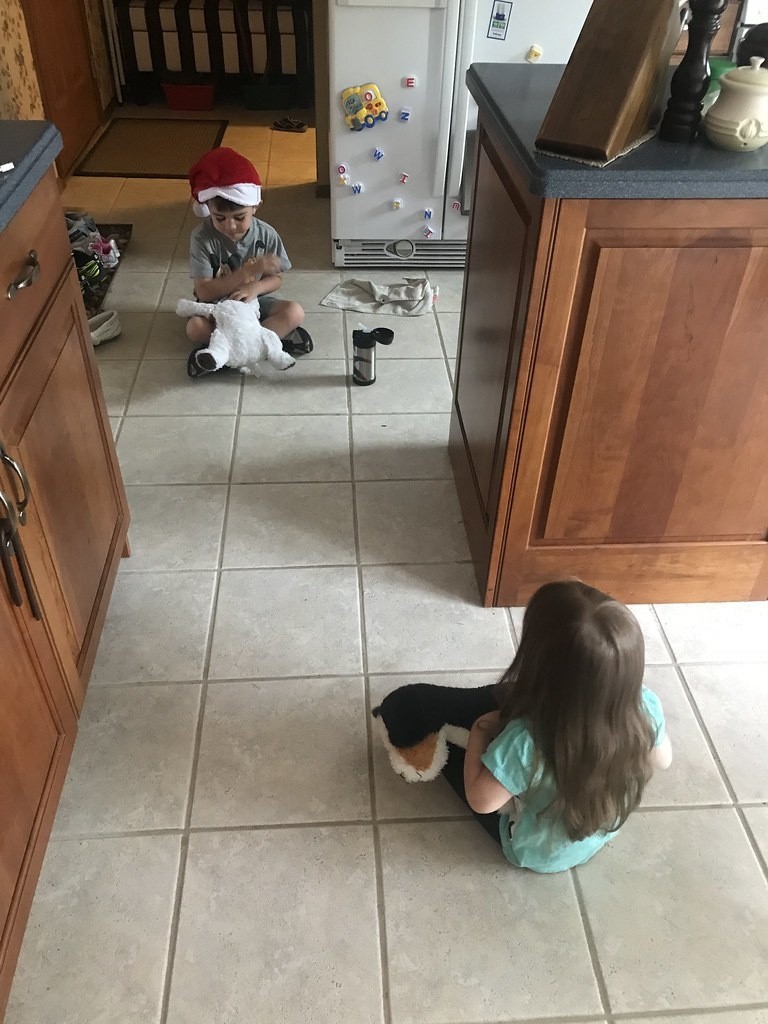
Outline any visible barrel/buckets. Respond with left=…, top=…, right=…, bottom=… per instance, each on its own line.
left=160, top=81, right=218, bottom=110
left=238, top=82, right=295, bottom=110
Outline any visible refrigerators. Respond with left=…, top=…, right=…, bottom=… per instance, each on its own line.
left=328, top=1, right=592, bottom=269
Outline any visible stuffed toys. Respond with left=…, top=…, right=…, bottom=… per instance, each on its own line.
left=174, top=278, right=296, bottom=376
left=370, top=683, right=501, bottom=784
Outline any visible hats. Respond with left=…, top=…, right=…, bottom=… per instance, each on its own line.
left=189, top=147, right=263, bottom=217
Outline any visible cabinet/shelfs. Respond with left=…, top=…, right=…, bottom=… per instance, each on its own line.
left=450, top=123, right=768, bottom=606
left=0, top=175, right=135, bottom=1020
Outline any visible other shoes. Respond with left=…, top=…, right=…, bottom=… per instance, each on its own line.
left=71, top=249, right=111, bottom=301
left=88, top=236, right=121, bottom=268
left=64, top=211, right=102, bottom=256
left=88, top=310, right=122, bottom=346
left=188, top=347, right=230, bottom=378
left=281, top=326, right=313, bottom=355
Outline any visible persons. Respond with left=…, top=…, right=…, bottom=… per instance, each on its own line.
left=185, top=146, right=313, bottom=377
left=443, top=580, right=673, bottom=873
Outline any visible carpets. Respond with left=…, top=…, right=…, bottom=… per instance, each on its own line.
left=77, top=117, right=229, bottom=180
left=82, top=223, right=134, bottom=322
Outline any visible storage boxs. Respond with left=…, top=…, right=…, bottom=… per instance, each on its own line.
left=241, top=72, right=304, bottom=109
left=157, top=74, right=217, bottom=111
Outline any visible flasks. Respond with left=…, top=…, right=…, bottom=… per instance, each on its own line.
left=353, top=322, right=394, bottom=384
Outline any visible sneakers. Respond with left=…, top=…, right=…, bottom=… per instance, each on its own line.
left=273, top=116, right=307, bottom=132
